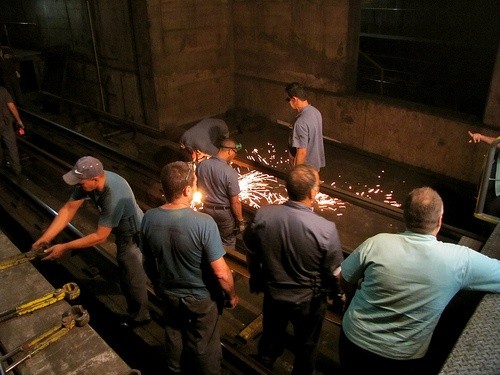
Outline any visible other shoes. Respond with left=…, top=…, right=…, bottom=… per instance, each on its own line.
left=119, top=318, right=144, bottom=330
left=248, top=353, right=274, bottom=370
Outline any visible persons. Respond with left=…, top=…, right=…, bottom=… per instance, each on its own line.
left=242, top=164, right=348, bottom=375
left=285, top=82, right=326, bottom=173
left=0, top=45, right=29, bottom=178
left=339, top=187, right=500, bottom=375
left=177, top=118, right=229, bottom=162
left=195, top=137, right=246, bottom=251
left=468, top=131, right=500, bottom=235
left=31, top=155, right=151, bottom=332
left=139, top=161, right=240, bottom=375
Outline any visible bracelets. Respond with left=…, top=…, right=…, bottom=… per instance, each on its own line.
left=238, top=220, right=245, bottom=226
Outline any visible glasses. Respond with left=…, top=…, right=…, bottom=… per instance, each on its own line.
left=286, top=96, right=294, bottom=102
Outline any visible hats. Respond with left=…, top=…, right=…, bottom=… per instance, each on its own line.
left=63, top=156, right=103, bottom=185
